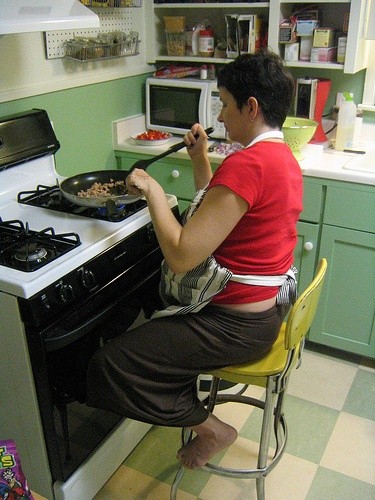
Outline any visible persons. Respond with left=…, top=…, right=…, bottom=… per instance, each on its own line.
left=75, top=52, right=302, bottom=471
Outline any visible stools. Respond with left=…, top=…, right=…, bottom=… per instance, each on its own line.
left=171, top=254, right=328, bottom=500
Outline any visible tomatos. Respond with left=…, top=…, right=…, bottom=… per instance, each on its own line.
left=137, top=130, right=171, bottom=140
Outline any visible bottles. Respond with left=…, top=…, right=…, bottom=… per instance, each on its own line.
left=186, top=18, right=215, bottom=58
left=66, top=30, right=139, bottom=60
left=334, top=91, right=357, bottom=149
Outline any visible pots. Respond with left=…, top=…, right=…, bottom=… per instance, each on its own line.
left=58, top=169, right=145, bottom=206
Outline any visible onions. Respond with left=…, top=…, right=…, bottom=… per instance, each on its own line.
left=215, top=142, right=243, bottom=156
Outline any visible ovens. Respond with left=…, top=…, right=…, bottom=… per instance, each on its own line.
left=0, top=192, right=197, bottom=500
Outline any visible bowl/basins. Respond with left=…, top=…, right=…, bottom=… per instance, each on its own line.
left=281, top=117, right=319, bottom=155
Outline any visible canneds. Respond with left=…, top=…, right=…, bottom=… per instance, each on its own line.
left=198, top=29, right=215, bottom=57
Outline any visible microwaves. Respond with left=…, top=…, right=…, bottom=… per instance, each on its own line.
left=144, top=73, right=233, bottom=142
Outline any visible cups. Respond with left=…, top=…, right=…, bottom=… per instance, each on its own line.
left=163, top=15, right=187, bottom=31
left=164, top=30, right=186, bottom=58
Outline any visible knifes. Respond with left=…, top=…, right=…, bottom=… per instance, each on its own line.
left=208, top=139, right=221, bottom=152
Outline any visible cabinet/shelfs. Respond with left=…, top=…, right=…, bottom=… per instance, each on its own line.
left=111, top=151, right=375, bottom=359
left=144, top=0, right=371, bottom=75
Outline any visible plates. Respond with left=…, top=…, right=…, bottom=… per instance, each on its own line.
left=130, top=131, right=173, bottom=146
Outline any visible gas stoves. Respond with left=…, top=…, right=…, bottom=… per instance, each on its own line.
left=0, top=106, right=178, bottom=300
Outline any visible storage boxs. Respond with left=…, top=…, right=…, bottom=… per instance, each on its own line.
left=279, top=9, right=349, bottom=64
